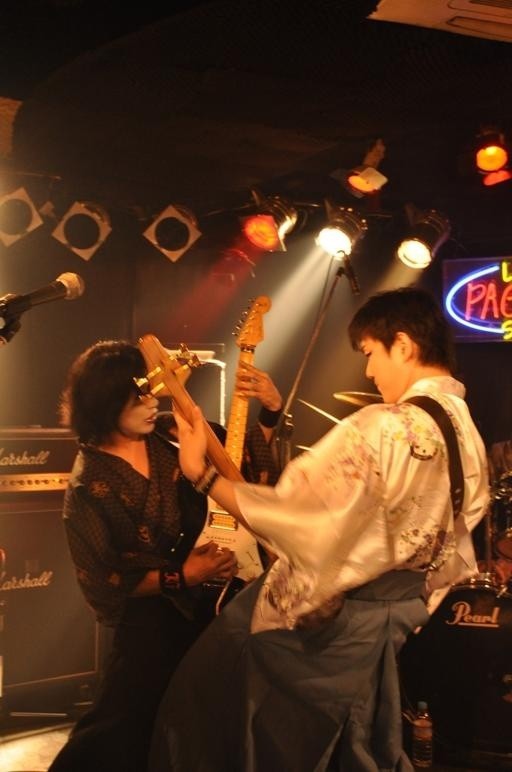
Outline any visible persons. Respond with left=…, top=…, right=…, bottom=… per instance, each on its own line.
left=142, top=286, right=490, bottom=772
left=44, top=337, right=285, bottom=772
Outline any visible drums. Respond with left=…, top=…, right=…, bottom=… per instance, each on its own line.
left=492, top=509, right=512, bottom=558
left=488, top=442, right=512, bottom=506
left=448, top=572, right=511, bottom=627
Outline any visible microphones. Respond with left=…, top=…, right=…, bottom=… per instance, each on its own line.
left=0, top=271, right=85, bottom=315
left=345, top=260, right=362, bottom=297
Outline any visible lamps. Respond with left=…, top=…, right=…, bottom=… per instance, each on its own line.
left=240, top=133, right=512, bottom=273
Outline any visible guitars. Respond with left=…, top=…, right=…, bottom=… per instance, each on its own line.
left=132, top=334, right=279, bottom=564
left=194, top=294, right=270, bottom=593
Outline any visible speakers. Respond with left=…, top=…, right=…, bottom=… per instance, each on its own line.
left=0, top=497, right=106, bottom=698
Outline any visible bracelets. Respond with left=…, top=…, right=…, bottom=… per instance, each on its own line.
left=159, top=565, right=187, bottom=591
left=257, top=405, right=283, bottom=429
left=191, top=467, right=219, bottom=494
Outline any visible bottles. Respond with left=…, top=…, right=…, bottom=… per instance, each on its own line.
left=410, top=702, right=434, bottom=769
